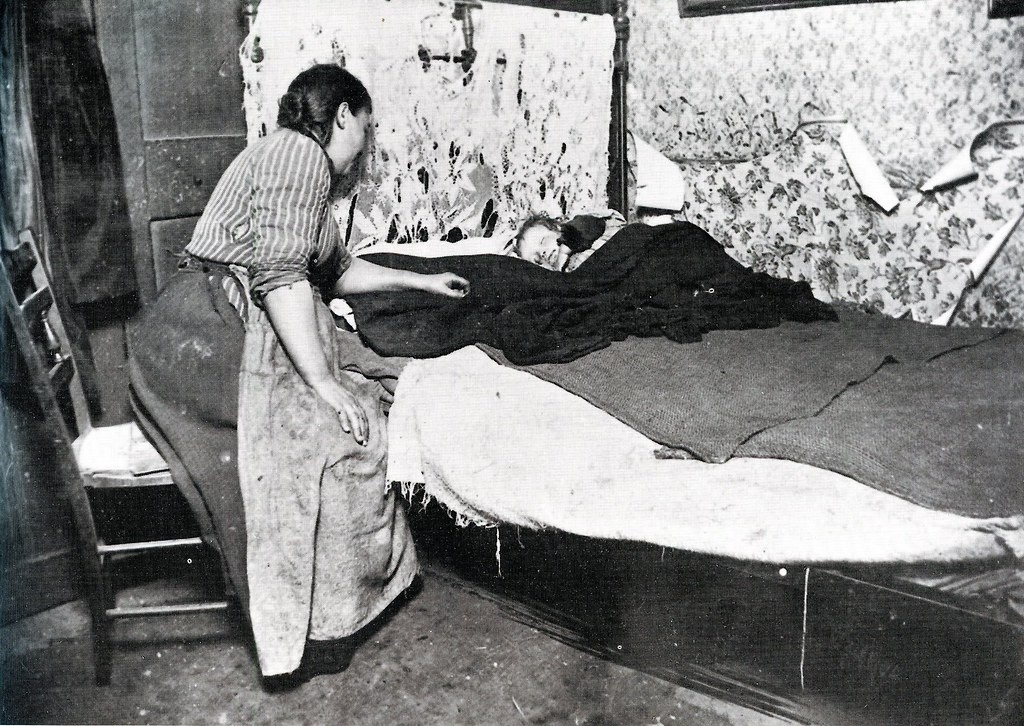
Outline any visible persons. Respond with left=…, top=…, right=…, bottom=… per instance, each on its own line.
left=503, top=208, right=815, bottom=331
left=127, top=62, right=470, bottom=670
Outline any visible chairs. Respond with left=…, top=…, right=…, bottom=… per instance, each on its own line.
left=0, top=229, right=297, bottom=684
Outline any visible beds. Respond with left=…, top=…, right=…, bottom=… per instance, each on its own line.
left=343, top=218, right=1024, bottom=726
